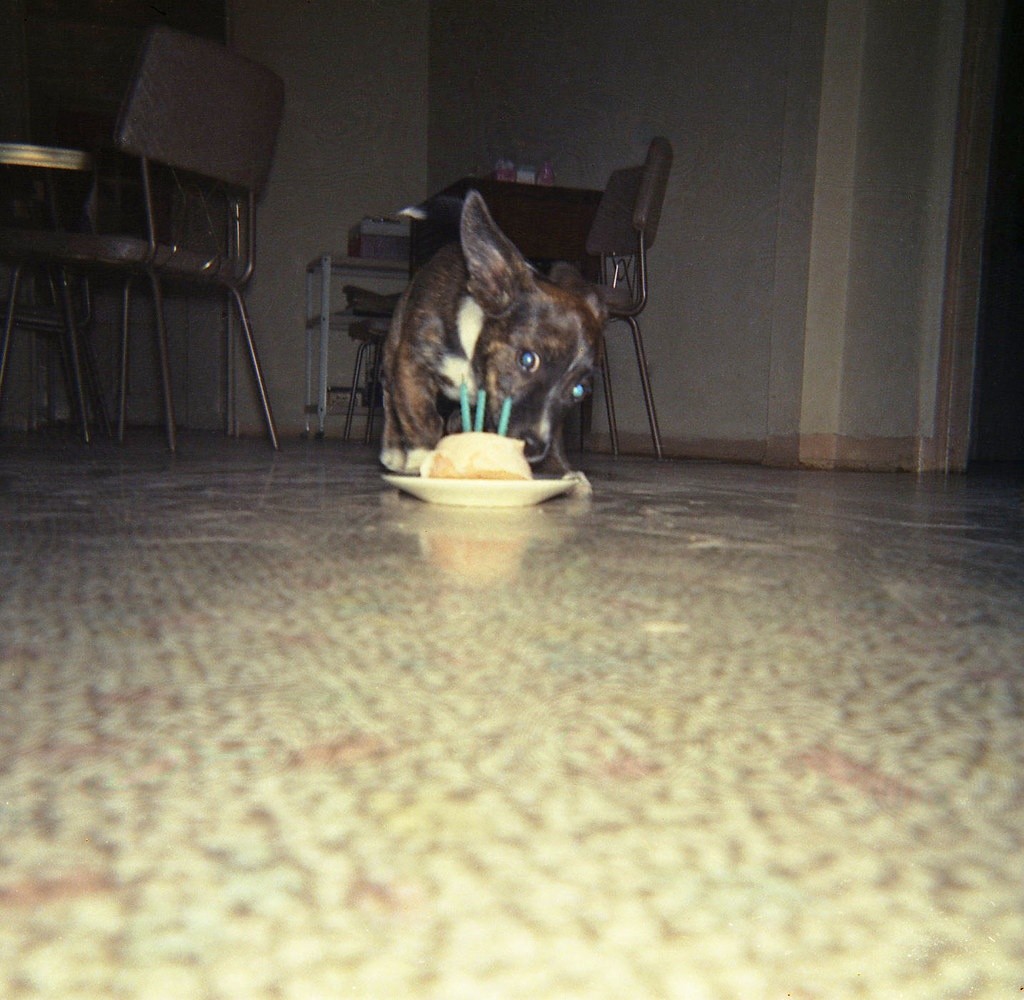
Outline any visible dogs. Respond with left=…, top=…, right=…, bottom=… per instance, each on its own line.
left=375, top=187, right=613, bottom=500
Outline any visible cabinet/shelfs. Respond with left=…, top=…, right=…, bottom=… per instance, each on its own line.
left=303, top=254, right=410, bottom=440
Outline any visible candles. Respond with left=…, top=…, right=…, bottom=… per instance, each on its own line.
left=459, top=374, right=473, bottom=432
left=473, top=386, right=488, bottom=432
left=497, top=392, right=513, bottom=437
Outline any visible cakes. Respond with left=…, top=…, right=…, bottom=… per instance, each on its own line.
left=424, top=432, right=534, bottom=482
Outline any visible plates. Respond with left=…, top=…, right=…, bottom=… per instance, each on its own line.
left=379, top=473, right=580, bottom=508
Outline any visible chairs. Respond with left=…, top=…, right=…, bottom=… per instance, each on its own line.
left=578, top=134, right=673, bottom=463
left=0, top=16, right=285, bottom=471
left=345, top=284, right=403, bottom=446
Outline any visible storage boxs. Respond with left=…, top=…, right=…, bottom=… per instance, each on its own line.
left=348, top=222, right=412, bottom=261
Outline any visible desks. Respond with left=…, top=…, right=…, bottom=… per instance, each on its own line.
left=411, top=176, right=605, bottom=452
left=0, top=143, right=109, bottom=451
